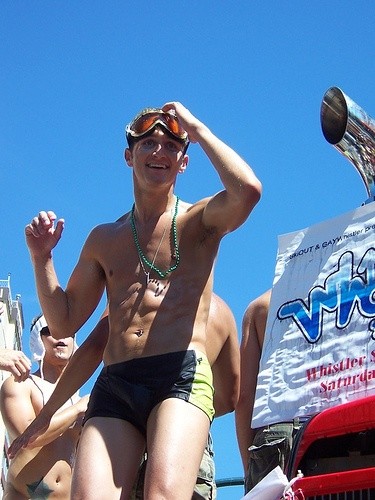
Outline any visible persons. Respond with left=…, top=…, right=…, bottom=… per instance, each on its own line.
left=0, top=349, right=31, bottom=383
left=235, top=288, right=313, bottom=500
left=0, top=313, right=91, bottom=500
left=5, top=292, right=240, bottom=500
left=25, top=102, right=264, bottom=500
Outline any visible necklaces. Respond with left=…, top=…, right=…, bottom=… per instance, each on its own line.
left=130, top=196, right=180, bottom=278
left=132, top=198, right=177, bottom=285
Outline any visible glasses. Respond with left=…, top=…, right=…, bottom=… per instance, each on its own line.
left=126, top=110, right=189, bottom=146
left=40, top=326, right=50, bottom=336
